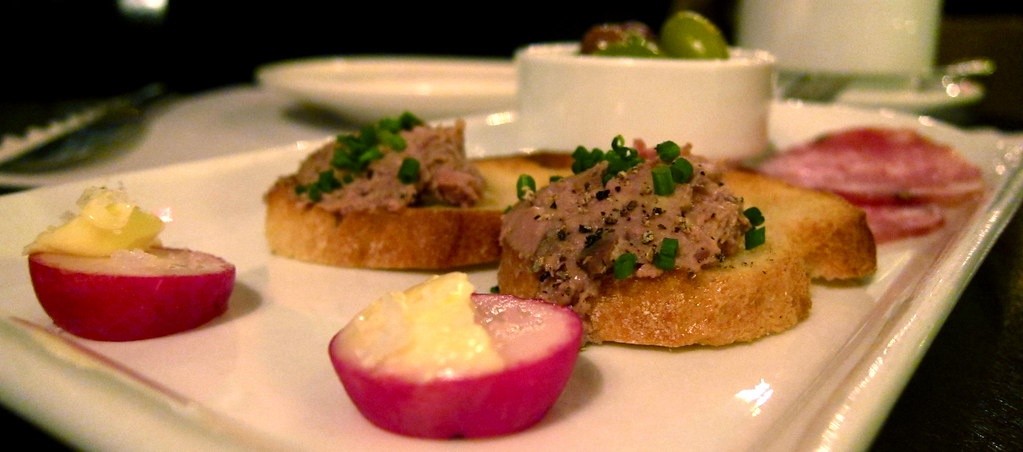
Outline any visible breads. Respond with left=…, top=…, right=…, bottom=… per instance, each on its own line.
left=262, top=151, right=877, bottom=346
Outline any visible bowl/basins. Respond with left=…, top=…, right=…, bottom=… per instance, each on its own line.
left=736, top=1, right=939, bottom=73
left=514, top=43, right=774, bottom=163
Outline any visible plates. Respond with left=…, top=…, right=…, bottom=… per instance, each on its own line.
left=258, top=55, right=515, bottom=126
left=835, top=82, right=981, bottom=109
left=0, top=113, right=1022, bottom=452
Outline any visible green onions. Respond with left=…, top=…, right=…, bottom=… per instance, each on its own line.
left=294, top=109, right=424, bottom=202
left=489, top=133, right=766, bottom=294
